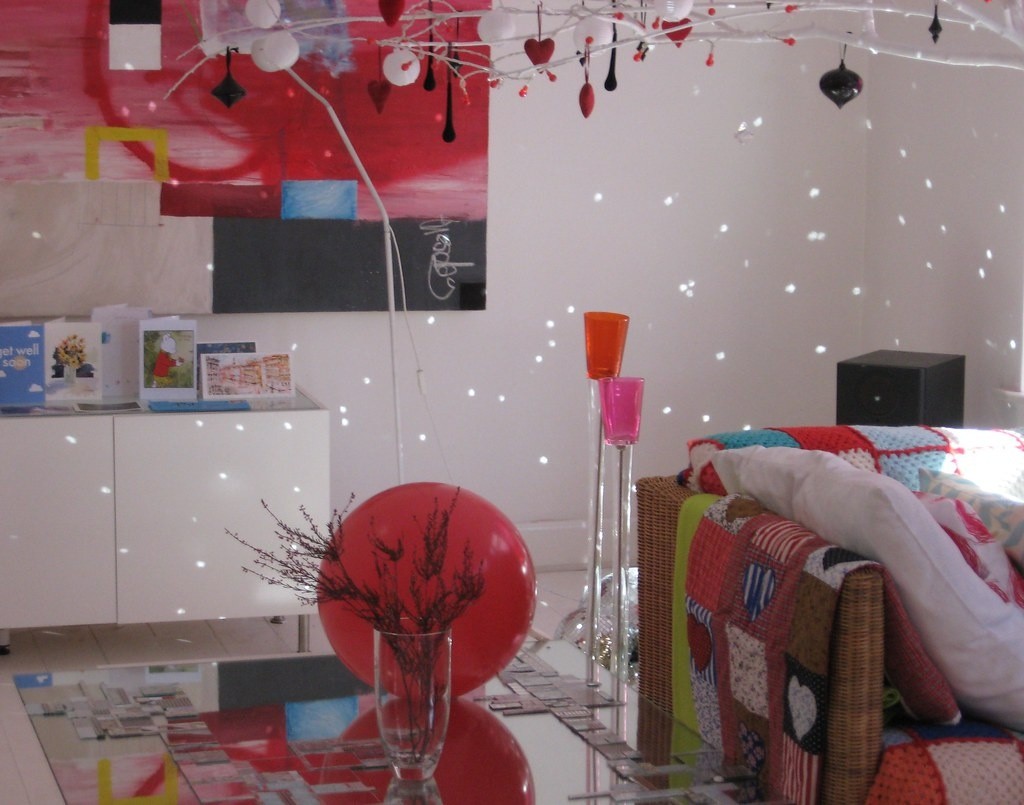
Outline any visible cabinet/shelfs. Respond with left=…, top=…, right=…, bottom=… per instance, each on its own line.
left=0, top=381, right=331, bottom=657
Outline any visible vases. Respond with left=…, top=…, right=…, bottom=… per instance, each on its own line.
left=373, top=618, right=451, bottom=779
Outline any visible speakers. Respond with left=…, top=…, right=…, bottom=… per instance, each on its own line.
left=836, top=349, right=965, bottom=430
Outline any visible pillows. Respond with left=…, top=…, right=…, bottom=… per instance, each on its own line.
left=913, top=490, right=1024, bottom=610
left=919, top=468, right=1024, bottom=568
left=710, top=445, right=1024, bottom=733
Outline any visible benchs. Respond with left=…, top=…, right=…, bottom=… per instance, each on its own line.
left=636, top=421, right=1024, bottom=805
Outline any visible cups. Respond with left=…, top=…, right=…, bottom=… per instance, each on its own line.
left=584, top=311, right=630, bottom=379
left=603, top=377, right=643, bottom=444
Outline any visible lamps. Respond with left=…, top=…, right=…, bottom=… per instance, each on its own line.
left=599, top=377, right=639, bottom=704
left=585, top=312, right=629, bottom=687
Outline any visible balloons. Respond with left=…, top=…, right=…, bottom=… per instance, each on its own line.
left=340, top=700, right=535, bottom=805
left=548, top=566, right=639, bottom=692
left=316, top=481, right=537, bottom=700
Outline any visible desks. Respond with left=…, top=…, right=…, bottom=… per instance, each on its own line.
left=0, top=659, right=443, bottom=805
